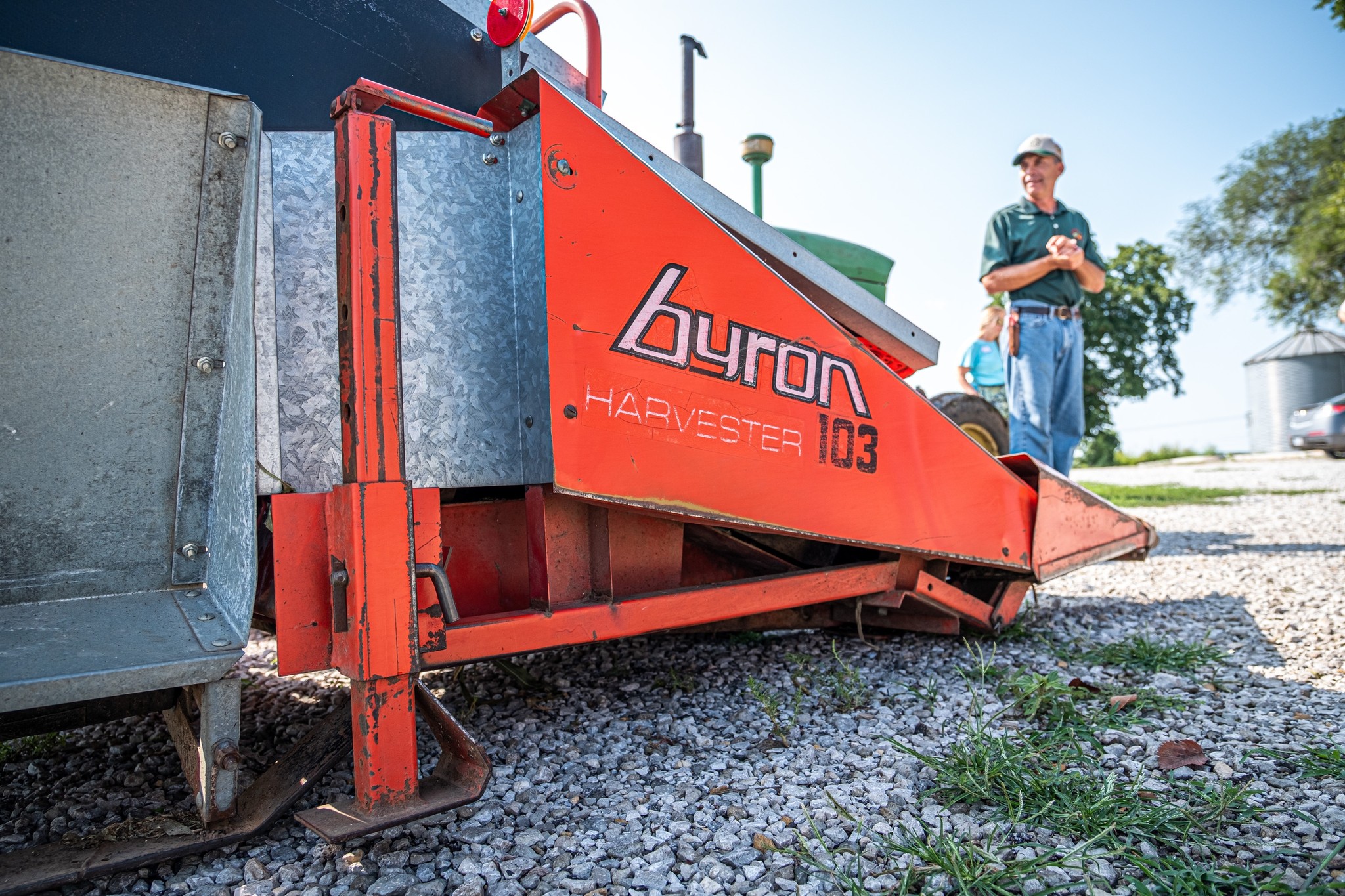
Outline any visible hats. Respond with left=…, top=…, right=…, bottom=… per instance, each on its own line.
left=1013, top=135, right=1062, bottom=166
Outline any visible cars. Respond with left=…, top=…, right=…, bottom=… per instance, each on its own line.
left=1288, top=392, right=1345, bottom=458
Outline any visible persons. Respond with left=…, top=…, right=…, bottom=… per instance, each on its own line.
left=977, top=136, right=1109, bottom=482
left=958, top=305, right=1010, bottom=423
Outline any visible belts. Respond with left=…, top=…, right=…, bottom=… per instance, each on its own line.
left=1019, top=307, right=1081, bottom=320
